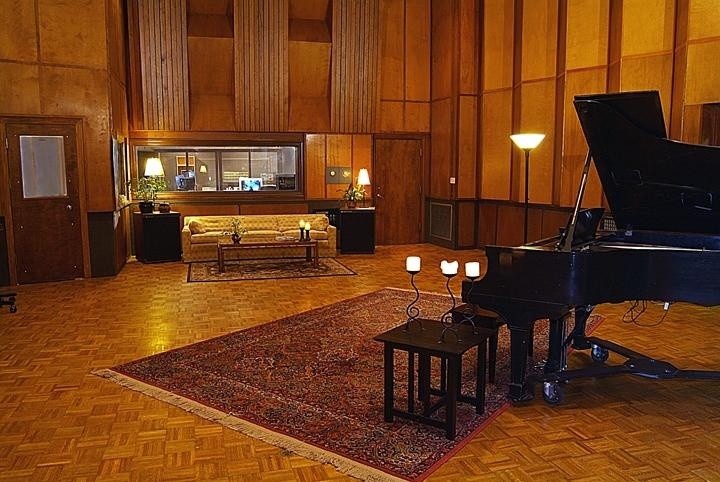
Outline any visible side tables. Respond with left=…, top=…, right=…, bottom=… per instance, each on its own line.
left=339, top=206, right=376, bottom=254
left=133, top=210, right=183, bottom=264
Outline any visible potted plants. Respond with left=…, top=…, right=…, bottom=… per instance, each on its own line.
left=336, top=182, right=368, bottom=207
left=220, top=218, right=249, bottom=245
left=126, top=175, right=171, bottom=213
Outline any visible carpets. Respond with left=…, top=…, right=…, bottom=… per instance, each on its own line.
left=187, top=257, right=357, bottom=283
left=90, top=286, right=606, bottom=482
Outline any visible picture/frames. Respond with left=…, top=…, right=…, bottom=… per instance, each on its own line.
left=239, top=177, right=262, bottom=191
left=276, top=174, right=299, bottom=192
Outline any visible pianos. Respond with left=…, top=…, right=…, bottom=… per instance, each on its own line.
left=462, top=90, right=719, bottom=403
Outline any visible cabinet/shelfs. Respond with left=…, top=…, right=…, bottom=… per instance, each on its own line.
left=176, top=156, right=195, bottom=177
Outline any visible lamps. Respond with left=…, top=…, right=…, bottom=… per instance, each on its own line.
left=357, top=168, right=371, bottom=208
left=510, top=133, right=547, bottom=244
left=143, top=158, right=164, bottom=176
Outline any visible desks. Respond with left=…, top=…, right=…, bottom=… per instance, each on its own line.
left=373, top=318, right=497, bottom=441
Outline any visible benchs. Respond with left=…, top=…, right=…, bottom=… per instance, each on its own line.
left=451, top=303, right=535, bottom=383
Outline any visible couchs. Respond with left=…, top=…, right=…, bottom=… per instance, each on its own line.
left=181, top=213, right=337, bottom=264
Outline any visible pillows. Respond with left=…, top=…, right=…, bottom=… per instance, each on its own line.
left=190, top=220, right=206, bottom=234
left=312, top=216, right=329, bottom=231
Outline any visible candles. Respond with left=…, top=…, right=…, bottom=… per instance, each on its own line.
left=299, top=219, right=305, bottom=228
left=405, top=256, right=421, bottom=271
left=305, top=223, right=311, bottom=230
left=440, top=260, right=459, bottom=274
left=464, top=261, right=480, bottom=277
